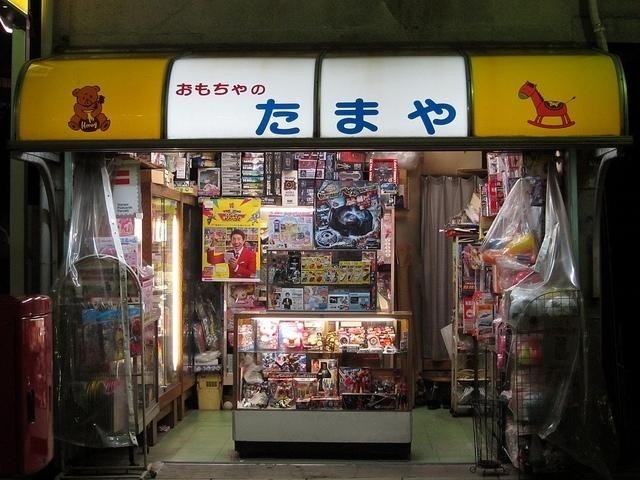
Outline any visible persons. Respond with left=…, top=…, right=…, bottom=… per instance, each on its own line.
left=282, top=293, right=292, bottom=309
left=207, top=229, right=256, bottom=278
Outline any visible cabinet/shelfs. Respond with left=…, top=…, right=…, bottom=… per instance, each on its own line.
left=87, top=183, right=202, bottom=445
left=233, top=310, right=413, bottom=459
left=447, top=204, right=586, bottom=479
